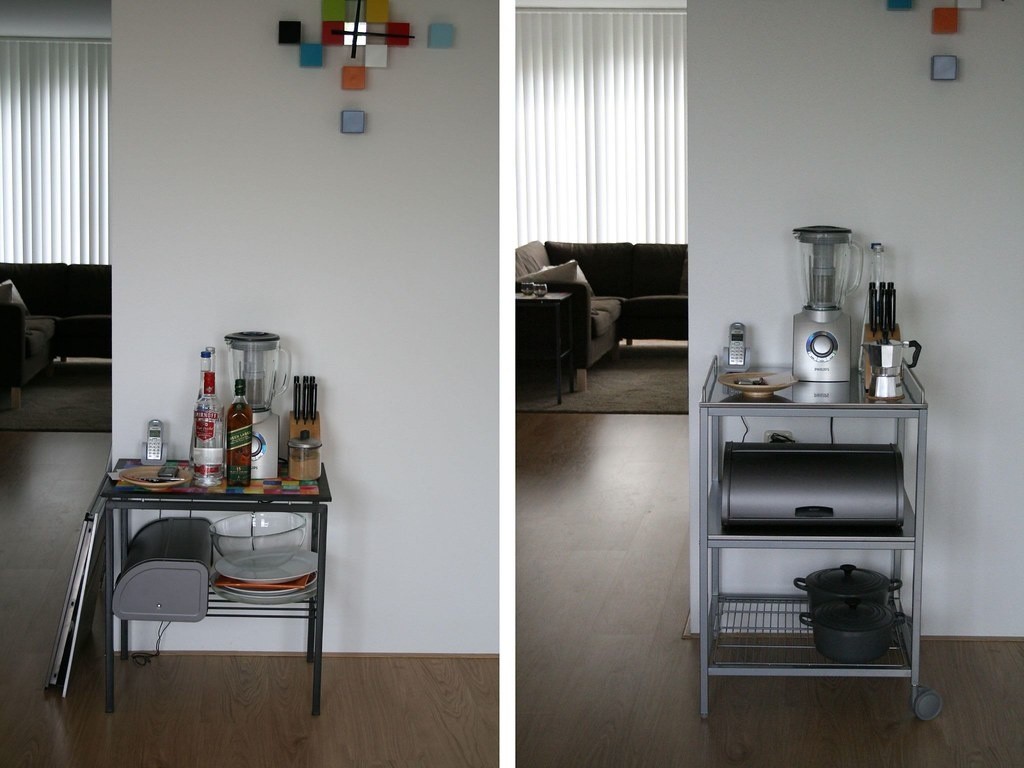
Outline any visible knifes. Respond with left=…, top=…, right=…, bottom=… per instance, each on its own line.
left=870, top=280, right=896, bottom=339
left=290, top=376, right=318, bottom=424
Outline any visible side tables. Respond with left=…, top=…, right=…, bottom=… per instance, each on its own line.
left=514, top=290, right=576, bottom=404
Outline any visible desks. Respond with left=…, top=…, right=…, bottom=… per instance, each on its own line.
left=97, top=457, right=333, bottom=717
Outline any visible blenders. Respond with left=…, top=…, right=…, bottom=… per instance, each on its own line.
left=790, top=225, right=863, bottom=384
left=224, top=332, right=290, bottom=478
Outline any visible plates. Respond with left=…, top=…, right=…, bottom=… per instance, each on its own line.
left=718, top=371, right=797, bottom=397
left=212, top=553, right=318, bottom=605
left=120, top=467, right=192, bottom=490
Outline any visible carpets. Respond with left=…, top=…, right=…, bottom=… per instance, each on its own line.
left=515, top=344, right=687, bottom=413
left=0, top=360, right=112, bottom=432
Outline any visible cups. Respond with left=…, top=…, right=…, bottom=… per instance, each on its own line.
left=534, top=284, right=547, bottom=297
left=287, top=431, right=321, bottom=481
left=521, top=282, right=534, bottom=296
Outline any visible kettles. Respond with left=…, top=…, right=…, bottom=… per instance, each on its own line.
left=859, top=333, right=921, bottom=400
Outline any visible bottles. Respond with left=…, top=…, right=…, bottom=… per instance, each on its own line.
left=858, top=245, right=885, bottom=368
left=226, top=379, right=253, bottom=488
left=187, top=347, right=224, bottom=486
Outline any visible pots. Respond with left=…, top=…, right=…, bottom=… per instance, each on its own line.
left=794, top=565, right=903, bottom=625
left=798, top=596, right=906, bottom=662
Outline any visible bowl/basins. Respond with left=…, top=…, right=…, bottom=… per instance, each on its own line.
left=209, top=513, right=307, bottom=569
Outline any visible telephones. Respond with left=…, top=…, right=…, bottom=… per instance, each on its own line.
left=141, top=419, right=168, bottom=466
left=722, top=322, right=751, bottom=372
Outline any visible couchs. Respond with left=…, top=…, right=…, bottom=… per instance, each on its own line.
left=0, top=261, right=111, bottom=410
left=514, top=238, right=689, bottom=392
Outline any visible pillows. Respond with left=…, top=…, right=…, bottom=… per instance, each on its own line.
left=517, top=259, right=577, bottom=285
left=0, top=278, right=29, bottom=316
left=0, top=283, right=13, bottom=303
left=542, top=259, right=594, bottom=298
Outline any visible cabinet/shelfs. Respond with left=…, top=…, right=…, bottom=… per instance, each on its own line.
left=697, top=355, right=931, bottom=717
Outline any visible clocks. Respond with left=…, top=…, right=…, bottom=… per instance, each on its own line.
left=275, top=0, right=455, bottom=135
